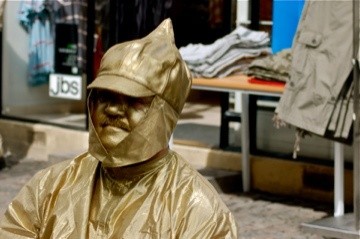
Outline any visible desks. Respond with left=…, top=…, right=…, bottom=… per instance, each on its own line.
left=190, top=75, right=354, bottom=216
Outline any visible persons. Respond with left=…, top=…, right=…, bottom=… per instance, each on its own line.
left=0, top=18, right=240, bottom=239
